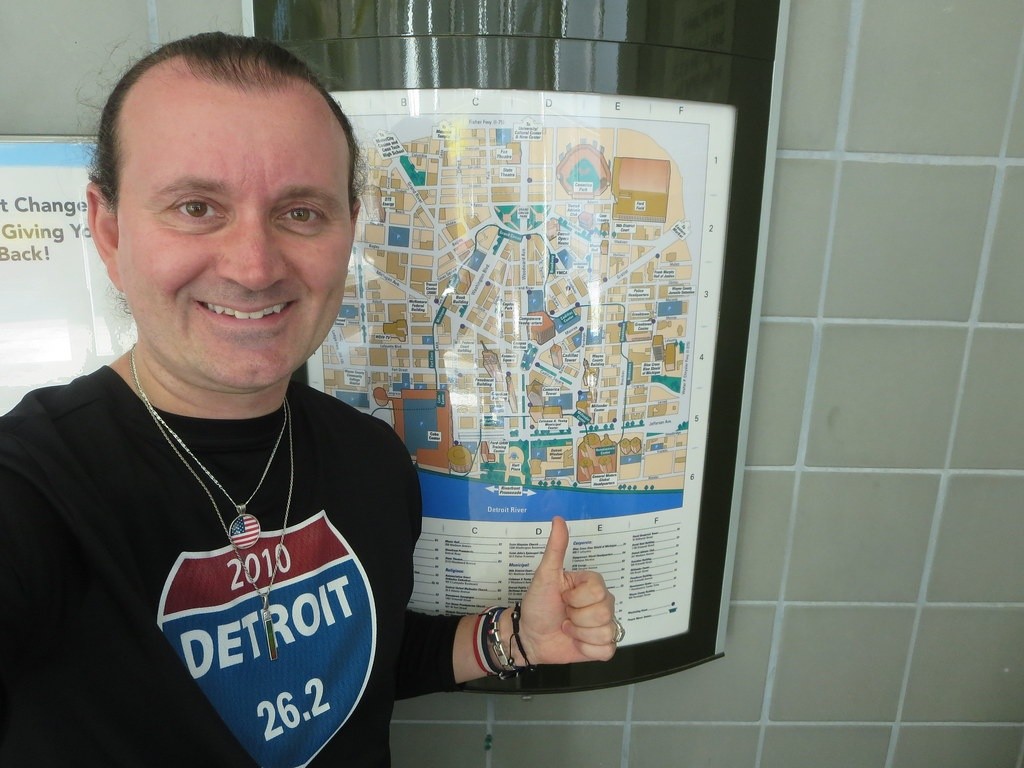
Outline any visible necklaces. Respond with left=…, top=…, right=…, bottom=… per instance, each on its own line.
left=129, top=345, right=293, bottom=662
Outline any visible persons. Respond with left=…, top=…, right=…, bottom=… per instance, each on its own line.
left=0, top=33, right=627, bottom=768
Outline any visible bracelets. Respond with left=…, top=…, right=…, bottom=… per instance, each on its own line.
left=472, top=601, right=536, bottom=680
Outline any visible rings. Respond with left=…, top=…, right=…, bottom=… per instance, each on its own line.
left=613, top=620, right=625, bottom=643
